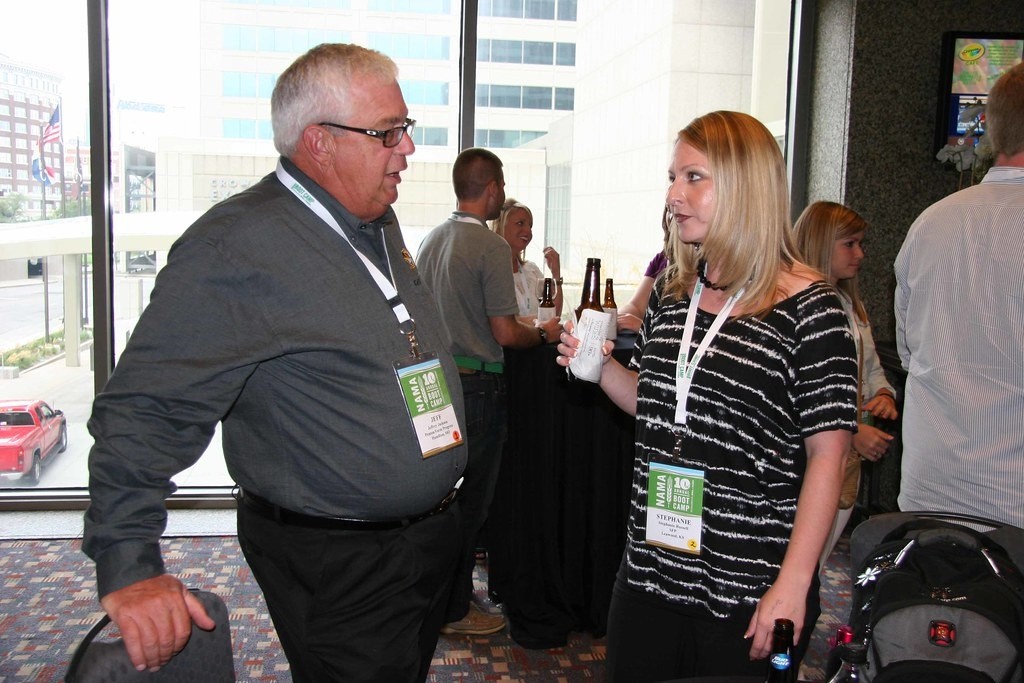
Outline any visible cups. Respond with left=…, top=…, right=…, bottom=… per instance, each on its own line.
left=536, top=278, right=557, bottom=303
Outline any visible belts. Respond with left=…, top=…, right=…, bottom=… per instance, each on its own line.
left=235, top=474, right=465, bottom=531
left=457, top=357, right=503, bottom=374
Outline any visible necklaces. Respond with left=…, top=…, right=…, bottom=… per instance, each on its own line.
left=695, top=254, right=750, bottom=290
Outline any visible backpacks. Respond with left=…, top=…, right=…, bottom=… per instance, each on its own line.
left=858, top=525, right=1024, bottom=683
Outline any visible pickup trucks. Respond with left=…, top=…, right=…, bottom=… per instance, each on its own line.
left=0, top=398, right=67, bottom=487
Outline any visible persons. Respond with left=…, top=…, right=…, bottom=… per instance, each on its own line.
left=416, top=146, right=899, bottom=635
left=892, top=60, right=1023, bottom=574
left=557, top=109, right=860, bottom=683
left=81, top=41, right=470, bottom=683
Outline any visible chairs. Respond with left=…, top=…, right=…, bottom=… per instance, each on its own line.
left=63, top=588, right=236, bottom=683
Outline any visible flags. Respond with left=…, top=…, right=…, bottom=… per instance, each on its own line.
left=37, top=105, right=60, bottom=147
left=75, top=144, right=84, bottom=198
left=32, top=147, right=58, bottom=187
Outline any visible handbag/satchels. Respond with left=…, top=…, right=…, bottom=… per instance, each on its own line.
left=837, top=450, right=861, bottom=510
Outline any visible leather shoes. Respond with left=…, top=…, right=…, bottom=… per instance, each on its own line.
left=440, top=598, right=506, bottom=634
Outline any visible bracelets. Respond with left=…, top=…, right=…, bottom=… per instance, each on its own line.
left=878, top=393, right=894, bottom=400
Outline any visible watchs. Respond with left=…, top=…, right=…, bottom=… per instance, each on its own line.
left=537, top=326, right=547, bottom=344
left=552, top=278, right=564, bottom=286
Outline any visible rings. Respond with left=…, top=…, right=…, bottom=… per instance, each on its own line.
left=873, top=451, right=879, bottom=457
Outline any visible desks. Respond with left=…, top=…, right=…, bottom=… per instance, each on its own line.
left=487, top=325, right=642, bottom=651
left=867, top=340, right=909, bottom=512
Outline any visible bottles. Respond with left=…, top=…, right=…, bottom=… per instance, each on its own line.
left=601, top=280, right=618, bottom=342
left=828, top=644, right=870, bottom=683
left=826, top=626, right=855, bottom=682
left=569, top=258, right=605, bottom=384
left=760, top=619, right=795, bottom=683
left=537, top=278, right=556, bottom=322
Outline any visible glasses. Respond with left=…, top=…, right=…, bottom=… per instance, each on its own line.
left=318, top=118, right=416, bottom=148
